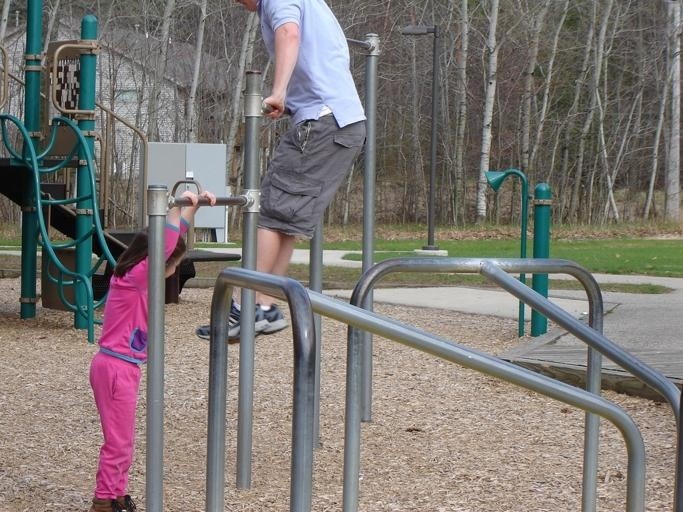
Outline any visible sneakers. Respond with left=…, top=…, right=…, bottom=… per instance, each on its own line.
left=195, top=298, right=271, bottom=341
left=228, top=303, right=289, bottom=345
left=89, top=494, right=137, bottom=512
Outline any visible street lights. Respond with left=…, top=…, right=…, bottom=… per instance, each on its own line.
left=398, top=22, right=442, bottom=250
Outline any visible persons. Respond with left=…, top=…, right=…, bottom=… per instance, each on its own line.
left=90, top=192, right=217, bottom=512
left=195, top=0, right=368, bottom=340
